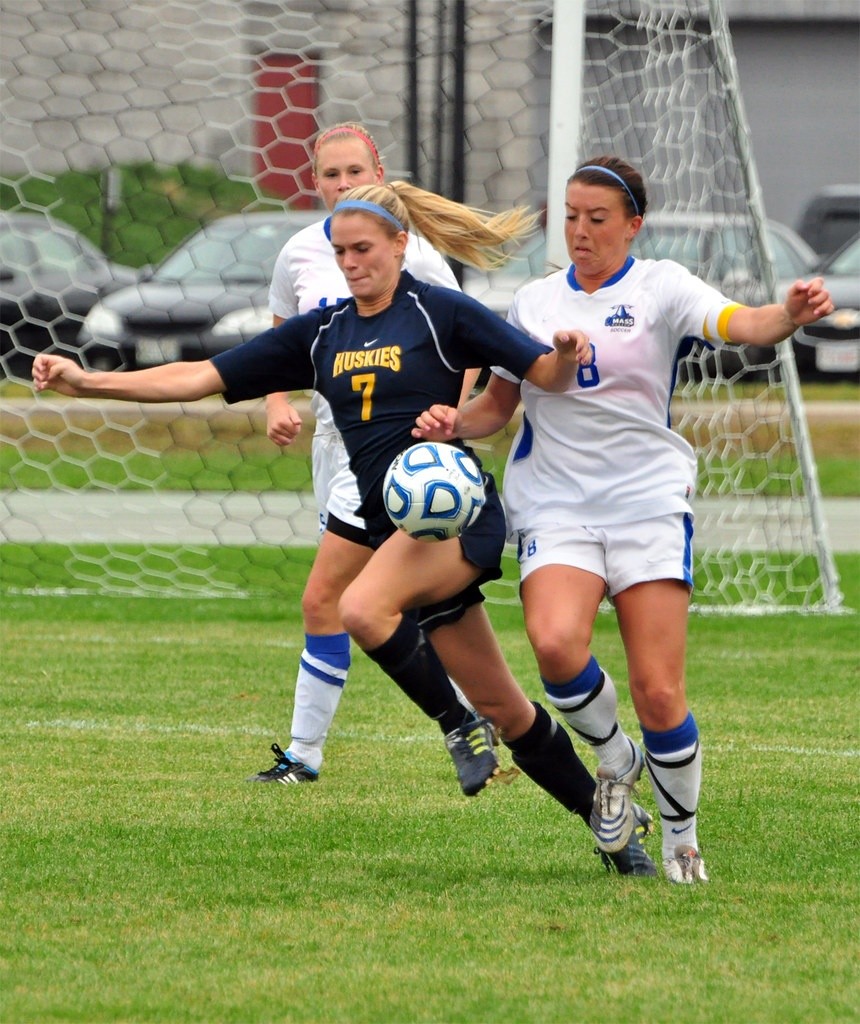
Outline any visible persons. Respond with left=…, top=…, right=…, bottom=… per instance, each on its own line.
left=30, top=181, right=658, bottom=877
left=250, top=122, right=503, bottom=797
left=411, top=155, right=833, bottom=882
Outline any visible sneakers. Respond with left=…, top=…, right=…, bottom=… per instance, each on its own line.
left=445, top=710, right=500, bottom=796
left=590, top=736, right=645, bottom=853
left=663, top=845, right=708, bottom=884
left=243, top=743, right=319, bottom=786
left=593, top=803, right=658, bottom=876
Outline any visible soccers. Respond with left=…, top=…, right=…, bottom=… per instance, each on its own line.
left=382, top=441, right=488, bottom=542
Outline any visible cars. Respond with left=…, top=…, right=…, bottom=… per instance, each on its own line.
left=466, top=212, right=819, bottom=385
left=775, top=231, right=859, bottom=381
left=76, top=209, right=335, bottom=374
left=0, top=211, right=142, bottom=382
